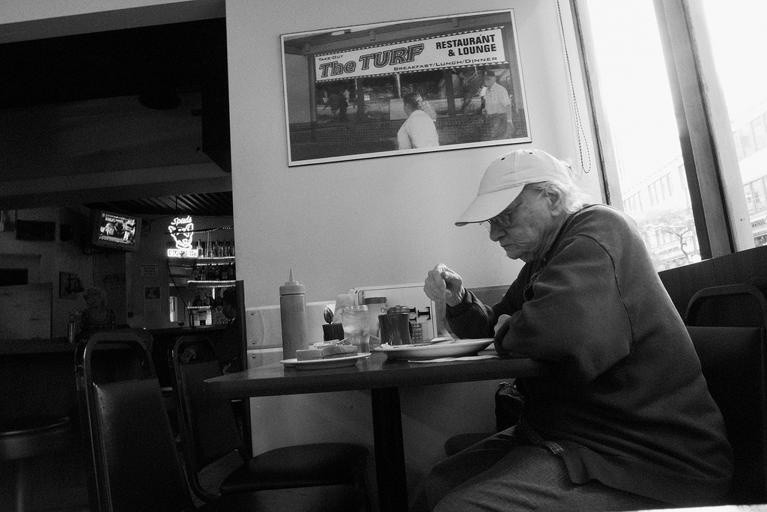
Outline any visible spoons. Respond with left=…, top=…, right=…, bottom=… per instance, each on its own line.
left=324, top=308, right=334, bottom=324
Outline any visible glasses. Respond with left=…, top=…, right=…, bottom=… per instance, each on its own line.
left=479, top=190, right=541, bottom=234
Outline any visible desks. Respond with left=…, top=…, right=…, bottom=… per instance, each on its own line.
left=0, top=399, right=81, bottom=511
left=204, top=351, right=535, bottom=512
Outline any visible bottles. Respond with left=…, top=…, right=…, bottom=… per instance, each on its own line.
left=278, top=267, right=310, bottom=370
left=363, top=296, right=387, bottom=336
left=193, top=236, right=236, bottom=280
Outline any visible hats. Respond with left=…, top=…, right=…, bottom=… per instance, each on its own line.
left=454, top=149, right=573, bottom=226
left=483, top=70, right=495, bottom=77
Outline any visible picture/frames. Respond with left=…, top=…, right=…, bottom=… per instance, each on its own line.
left=279, top=9, right=532, bottom=167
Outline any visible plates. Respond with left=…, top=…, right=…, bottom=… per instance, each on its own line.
left=371, top=337, right=496, bottom=360
left=278, top=352, right=372, bottom=370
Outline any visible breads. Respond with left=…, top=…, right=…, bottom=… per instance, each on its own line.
left=321, top=346, right=356, bottom=359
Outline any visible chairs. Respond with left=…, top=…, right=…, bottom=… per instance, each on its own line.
left=681, top=283, right=766, bottom=433
left=82, top=331, right=214, bottom=512
left=170, top=334, right=371, bottom=502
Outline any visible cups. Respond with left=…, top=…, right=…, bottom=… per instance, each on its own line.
left=340, top=303, right=371, bottom=356
left=378, top=312, right=412, bottom=347
left=321, top=322, right=346, bottom=343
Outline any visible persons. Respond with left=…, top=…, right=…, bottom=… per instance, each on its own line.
left=404, top=147, right=737, bottom=512
left=394, top=91, right=440, bottom=153
left=476, top=68, right=517, bottom=137
